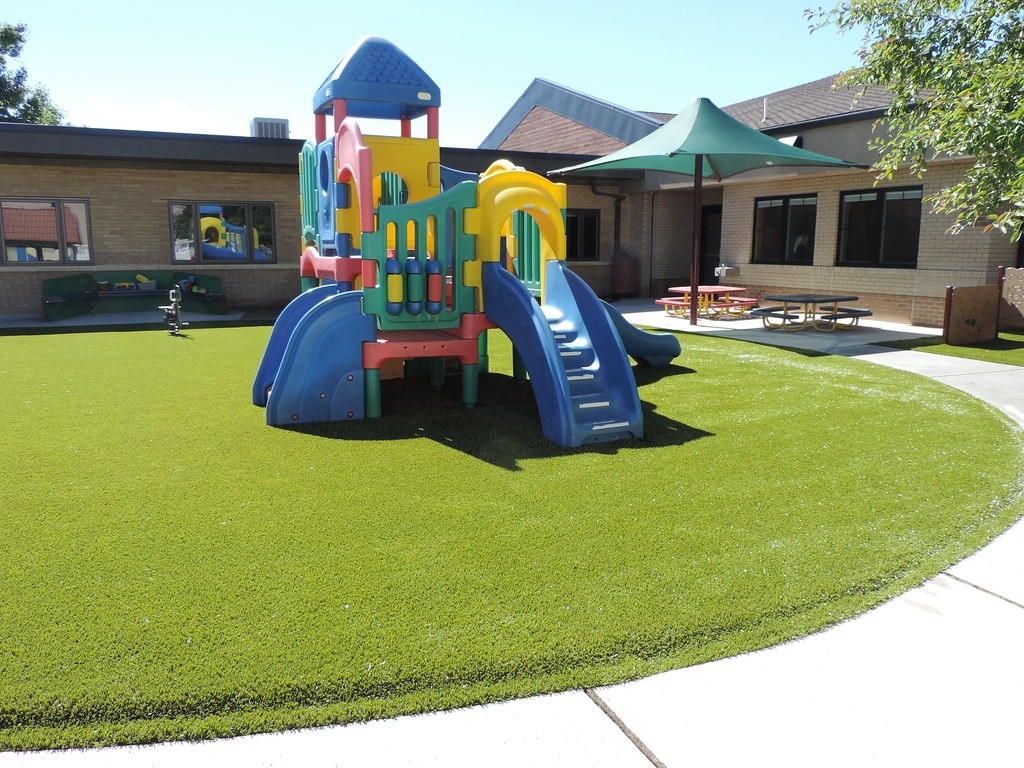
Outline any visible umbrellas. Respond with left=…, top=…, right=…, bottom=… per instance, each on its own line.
left=546, top=98, right=869, bottom=325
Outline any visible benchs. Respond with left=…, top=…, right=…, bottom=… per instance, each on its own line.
left=654, top=285, right=758, bottom=320
left=41, top=270, right=234, bottom=323
left=749, top=292, right=873, bottom=334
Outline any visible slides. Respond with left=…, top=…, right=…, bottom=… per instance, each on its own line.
left=599, top=299, right=681, bottom=364
left=201, top=243, right=271, bottom=260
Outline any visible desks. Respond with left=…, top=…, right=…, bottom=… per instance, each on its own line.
left=668, top=286, right=746, bottom=304
left=765, top=294, right=859, bottom=314
